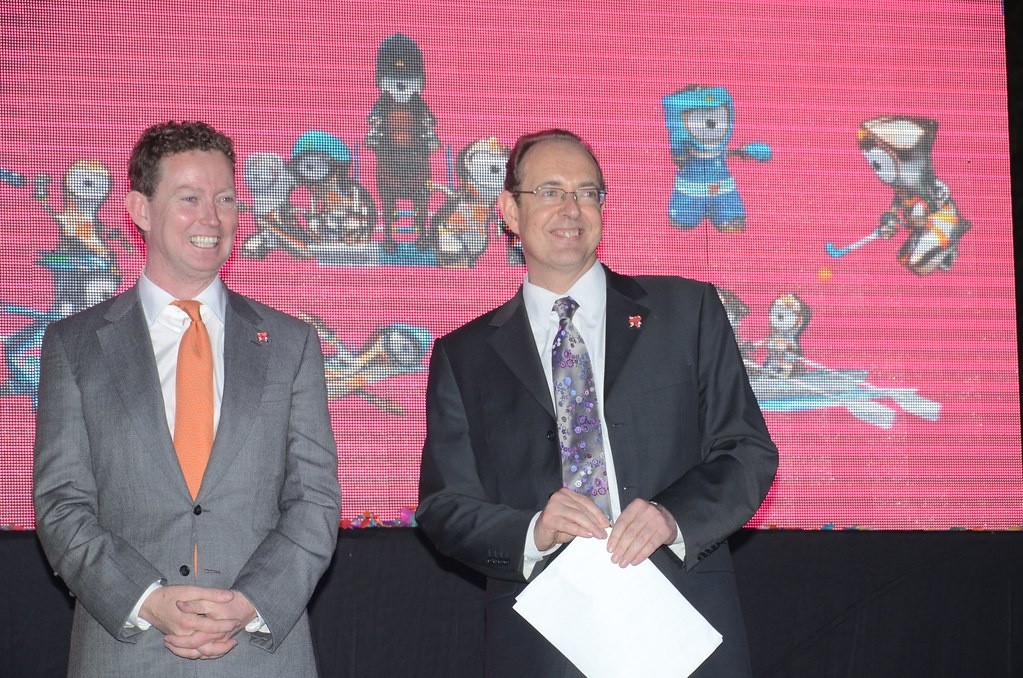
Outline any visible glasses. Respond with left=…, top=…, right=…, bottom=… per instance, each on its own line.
left=513, top=187, right=608, bottom=206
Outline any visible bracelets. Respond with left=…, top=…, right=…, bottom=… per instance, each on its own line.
left=649, top=501, right=658, bottom=505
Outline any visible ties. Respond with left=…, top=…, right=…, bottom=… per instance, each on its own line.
left=551, top=296, right=612, bottom=529
left=171, top=300, right=212, bottom=580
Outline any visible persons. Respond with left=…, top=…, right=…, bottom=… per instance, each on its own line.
left=415, top=130, right=780, bottom=678
left=32, top=121, right=341, bottom=678
